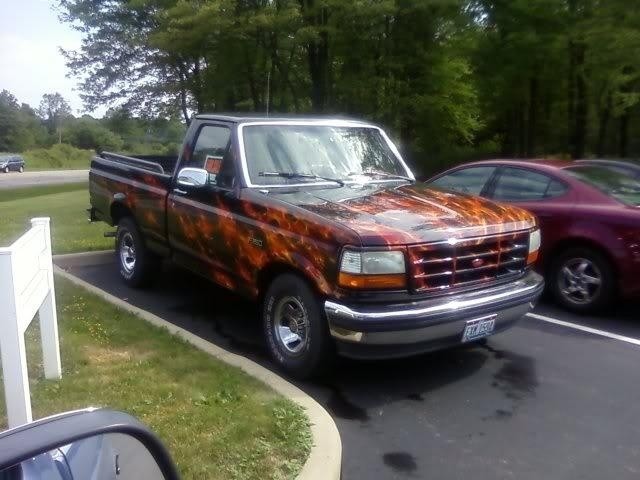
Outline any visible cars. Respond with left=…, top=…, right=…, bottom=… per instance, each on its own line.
left=418, top=157, right=640, bottom=316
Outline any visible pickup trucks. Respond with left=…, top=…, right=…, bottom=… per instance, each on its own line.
left=86, top=112, right=545, bottom=382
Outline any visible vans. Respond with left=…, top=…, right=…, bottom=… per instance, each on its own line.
left=0, top=154, right=26, bottom=175
